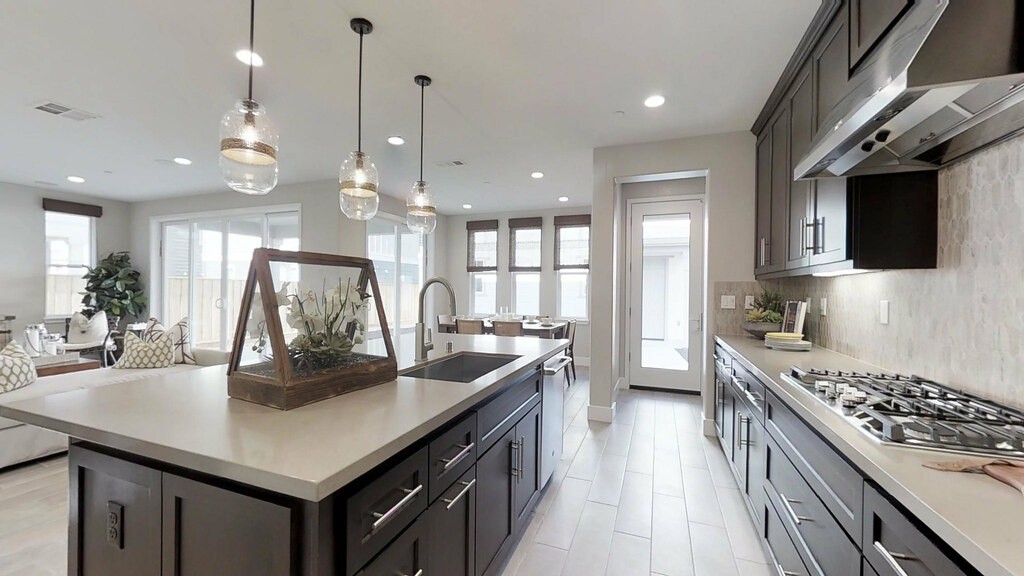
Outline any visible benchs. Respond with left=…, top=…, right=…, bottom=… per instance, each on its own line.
left=0, top=348, right=231, bottom=474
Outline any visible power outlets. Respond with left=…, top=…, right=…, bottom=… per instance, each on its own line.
left=106, top=500, right=125, bottom=550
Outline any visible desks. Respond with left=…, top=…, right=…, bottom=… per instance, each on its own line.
left=447, top=319, right=566, bottom=339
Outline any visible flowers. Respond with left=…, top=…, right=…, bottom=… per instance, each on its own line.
left=251, top=277, right=372, bottom=371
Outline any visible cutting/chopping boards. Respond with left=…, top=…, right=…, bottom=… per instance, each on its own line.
left=982, top=464, right=1024, bottom=497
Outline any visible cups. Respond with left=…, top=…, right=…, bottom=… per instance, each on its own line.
left=457, top=315, right=464, bottom=319
left=528, top=315, right=536, bottom=321
left=503, top=313, right=513, bottom=319
left=543, top=318, right=552, bottom=324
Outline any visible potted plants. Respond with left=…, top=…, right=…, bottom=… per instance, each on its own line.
left=78, top=250, right=147, bottom=366
left=743, top=280, right=793, bottom=340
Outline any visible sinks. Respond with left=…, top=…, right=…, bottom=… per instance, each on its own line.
left=396, top=350, right=526, bottom=384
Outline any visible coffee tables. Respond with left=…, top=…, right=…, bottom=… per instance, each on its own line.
left=35, top=358, right=102, bottom=378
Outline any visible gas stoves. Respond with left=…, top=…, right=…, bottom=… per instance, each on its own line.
left=782, top=368, right=1024, bottom=460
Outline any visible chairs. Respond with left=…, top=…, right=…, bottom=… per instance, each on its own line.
left=492, top=320, right=524, bottom=337
left=523, top=315, right=549, bottom=321
left=437, top=314, right=452, bottom=324
left=456, top=319, right=484, bottom=334
left=57, top=314, right=120, bottom=368
left=564, top=318, right=577, bottom=386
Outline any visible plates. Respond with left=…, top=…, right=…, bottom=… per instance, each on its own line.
left=765, top=333, right=810, bottom=350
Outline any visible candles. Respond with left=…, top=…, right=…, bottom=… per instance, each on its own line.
left=505, top=306, right=509, bottom=315
left=499, top=306, right=502, bottom=316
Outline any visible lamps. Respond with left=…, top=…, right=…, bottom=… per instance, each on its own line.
left=407, top=75, right=437, bottom=235
left=218, top=0, right=279, bottom=195
left=339, top=18, right=380, bottom=221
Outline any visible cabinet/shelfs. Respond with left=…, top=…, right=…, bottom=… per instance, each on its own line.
left=784, top=0, right=938, bottom=276
left=712, top=343, right=983, bottom=576
left=476, top=364, right=542, bottom=576
left=750, top=69, right=785, bottom=281
left=320, top=406, right=476, bottom=576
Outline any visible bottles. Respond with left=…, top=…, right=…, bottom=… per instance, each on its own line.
left=25, top=324, right=47, bottom=358
left=40, top=334, right=65, bottom=358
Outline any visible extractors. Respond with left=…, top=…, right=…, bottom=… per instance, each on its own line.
left=793, top=0, right=1024, bottom=179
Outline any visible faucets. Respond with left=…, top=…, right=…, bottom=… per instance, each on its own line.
left=414, top=276, right=457, bottom=362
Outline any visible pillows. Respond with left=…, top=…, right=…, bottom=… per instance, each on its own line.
left=67, top=311, right=114, bottom=347
left=0, top=340, right=39, bottom=394
left=144, top=316, right=197, bottom=365
left=112, top=331, right=175, bottom=369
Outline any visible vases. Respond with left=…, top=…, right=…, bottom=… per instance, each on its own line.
left=742, top=321, right=781, bottom=339
